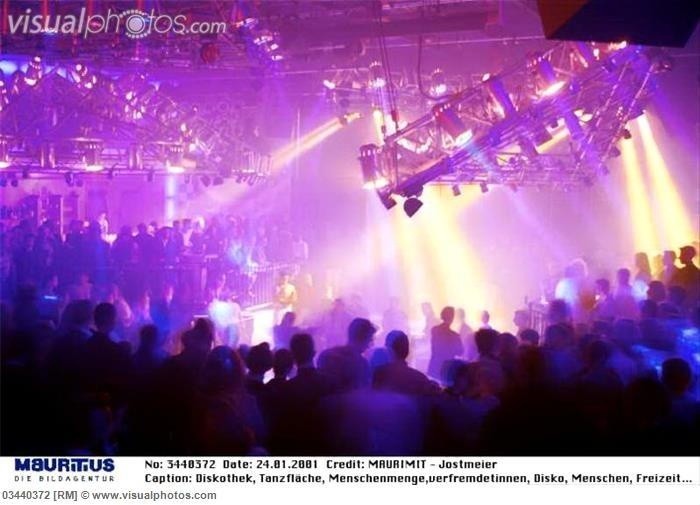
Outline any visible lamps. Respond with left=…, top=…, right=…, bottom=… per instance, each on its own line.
left=0, top=161, right=271, bottom=192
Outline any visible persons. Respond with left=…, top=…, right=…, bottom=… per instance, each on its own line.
left=0, top=208, right=700, bottom=454
